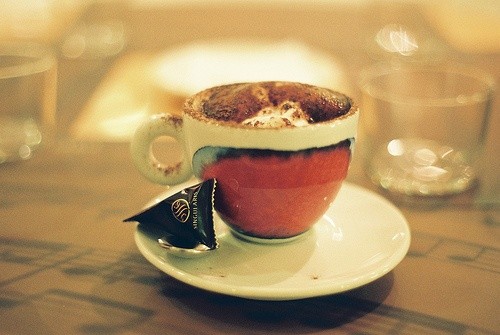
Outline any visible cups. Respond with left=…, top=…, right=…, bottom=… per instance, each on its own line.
left=132, top=79, right=360, bottom=244
left=0, top=39, right=58, bottom=163
left=358, top=60, right=493, bottom=204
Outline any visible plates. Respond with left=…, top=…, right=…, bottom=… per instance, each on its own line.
left=133, top=179, right=412, bottom=302
